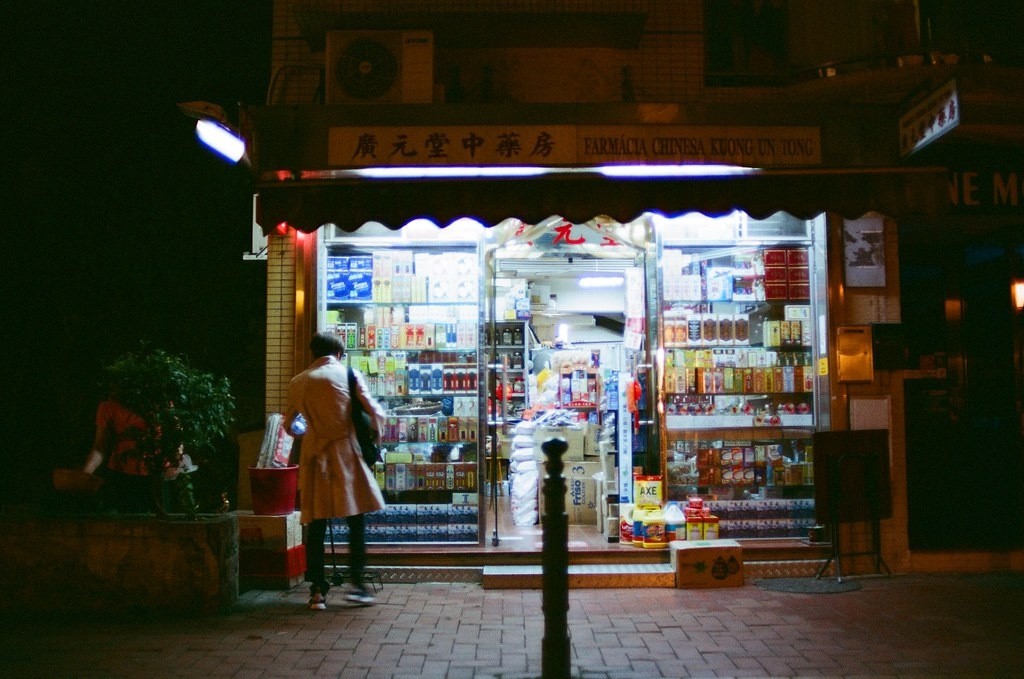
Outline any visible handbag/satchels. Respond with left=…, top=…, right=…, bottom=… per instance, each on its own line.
left=348, top=367, right=384, bottom=466
left=537, top=367, right=554, bottom=392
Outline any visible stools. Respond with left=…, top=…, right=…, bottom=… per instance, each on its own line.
left=486, top=455, right=505, bottom=497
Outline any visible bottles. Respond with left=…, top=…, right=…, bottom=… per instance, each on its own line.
left=608, top=375, right=619, bottom=407
left=291, top=412, right=307, bottom=434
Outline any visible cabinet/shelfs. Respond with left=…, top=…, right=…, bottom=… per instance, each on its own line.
left=666, top=297, right=815, bottom=489
left=486, top=321, right=530, bottom=425
left=326, top=299, right=478, bottom=543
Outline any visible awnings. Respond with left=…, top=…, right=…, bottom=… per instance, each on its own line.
left=256, top=166, right=954, bottom=224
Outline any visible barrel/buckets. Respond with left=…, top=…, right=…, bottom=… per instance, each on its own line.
left=246, top=462, right=300, bottom=515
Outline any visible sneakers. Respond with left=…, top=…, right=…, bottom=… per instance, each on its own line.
left=343, top=584, right=374, bottom=605
left=306, top=590, right=326, bottom=609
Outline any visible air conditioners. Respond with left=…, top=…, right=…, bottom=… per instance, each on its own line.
left=324, top=30, right=434, bottom=105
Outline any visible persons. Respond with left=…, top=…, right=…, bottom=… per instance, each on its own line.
left=68, top=390, right=181, bottom=518
left=284, top=331, right=386, bottom=610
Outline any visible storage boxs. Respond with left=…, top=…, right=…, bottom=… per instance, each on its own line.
left=325, top=251, right=478, bottom=542
left=665, top=249, right=817, bottom=589
left=235, top=510, right=304, bottom=590
left=536, top=369, right=621, bottom=537
